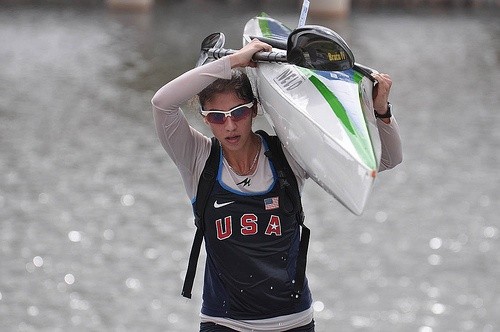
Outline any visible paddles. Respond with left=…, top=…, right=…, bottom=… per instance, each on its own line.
left=194, top=25, right=355, bottom=72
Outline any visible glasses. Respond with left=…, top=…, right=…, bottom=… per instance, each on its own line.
left=199, top=100, right=255, bottom=124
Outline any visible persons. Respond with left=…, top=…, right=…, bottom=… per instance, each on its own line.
left=151, top=37, right=403, bottom=332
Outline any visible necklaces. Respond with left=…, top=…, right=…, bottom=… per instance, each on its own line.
left=221, top=136, right=261, bottom=176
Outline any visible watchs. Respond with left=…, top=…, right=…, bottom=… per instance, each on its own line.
left=374, top=102, right=392, bottom=119
